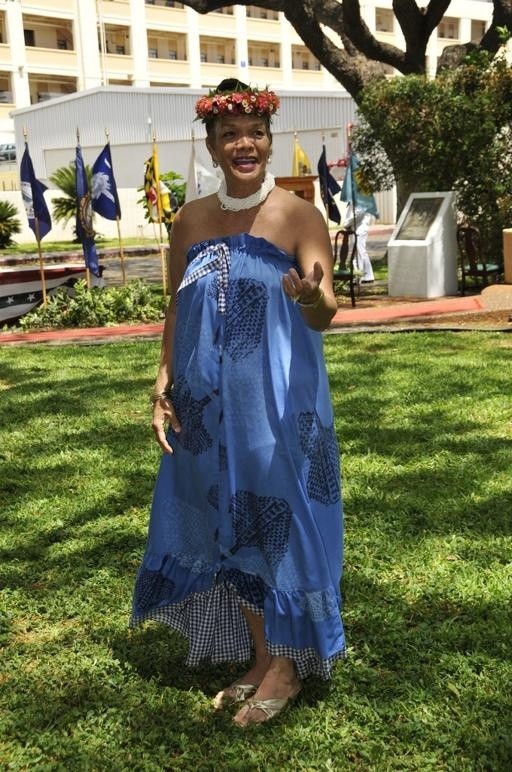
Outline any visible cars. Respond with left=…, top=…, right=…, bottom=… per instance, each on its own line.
left=0, top=144, right=16, bottom=162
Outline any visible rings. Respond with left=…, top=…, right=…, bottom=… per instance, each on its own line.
left=290, top=295, right=301, bottom=305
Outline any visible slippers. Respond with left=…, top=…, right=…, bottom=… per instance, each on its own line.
left=234, top=684, right=304, bottom=728
left=212, top=677, right=257, bottom=709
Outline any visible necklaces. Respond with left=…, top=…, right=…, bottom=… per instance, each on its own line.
left=217, top=170, right=275, bottom=211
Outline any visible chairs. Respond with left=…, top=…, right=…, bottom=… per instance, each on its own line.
left=327, top=226, right=361, bottom=308
left=456, top=222, right=501, bottom=297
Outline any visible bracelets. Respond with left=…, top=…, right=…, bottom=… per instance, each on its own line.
left=149, top=392, right=170, bottom=403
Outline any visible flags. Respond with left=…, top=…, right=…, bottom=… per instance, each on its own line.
left=317, top=144, right=343, bottom=225
left=90, top=142, right=121, bottom=221
left=341, top=143, right=380, bottom=218
left=144, top=142, right=178, bottom=225
left=293, top=142, right=312, bottom=176
left=75, top=144, right=99, bottom=277
left=19, top=142, right=51, bottom=242
left=184, top=143, right=220, bottom=205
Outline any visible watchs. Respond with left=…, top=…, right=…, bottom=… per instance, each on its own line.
left=298, top=292, right=325, bottom=309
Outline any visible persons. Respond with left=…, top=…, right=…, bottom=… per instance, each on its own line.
left=344, top=204, right=375, bottom=284
left=124, top=77, right=347, bottom=733
